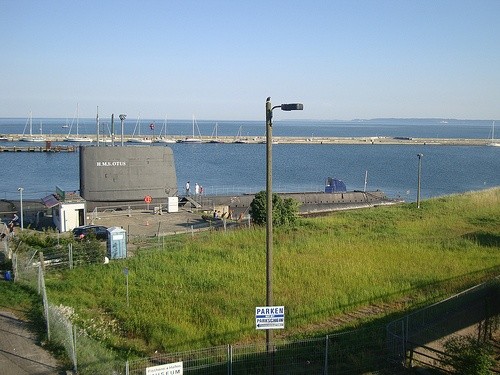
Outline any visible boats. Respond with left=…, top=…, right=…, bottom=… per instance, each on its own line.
left=262, top=139, right=279, bottom=145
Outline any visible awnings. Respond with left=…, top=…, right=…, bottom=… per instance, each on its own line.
left=42, top=195, right=60, bottom=209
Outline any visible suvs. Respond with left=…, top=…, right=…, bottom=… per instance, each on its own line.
left=71, top=224, right=110, bottom=243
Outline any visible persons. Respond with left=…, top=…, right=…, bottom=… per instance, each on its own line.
left=186, top=182, right=189, bottom=196
left=10, top=223, right=14, bottom=236
left=200, top=186, right=203, bottom=194
left=195, top=183, right=199, bottom=193
left=13, top=214, right=19, bottom=225
left=214, top=210, right=219, bottom=219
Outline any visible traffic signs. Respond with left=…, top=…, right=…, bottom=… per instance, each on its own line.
left=255, top=306, right=285, bottom=330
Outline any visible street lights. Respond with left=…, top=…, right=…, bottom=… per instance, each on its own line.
left=119, top=114, right=127, bottom=147
left=17, top=187, right=25, bottom=231
left=264, top=97, right=304, bottom=375
left=416, top=152, right=424, bottom=209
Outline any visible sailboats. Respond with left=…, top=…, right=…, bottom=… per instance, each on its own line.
left=151, top=113, right=177, bottom=144
left=232, top=126, right=249, bottom=144
left=127, top=110, right=153, bottom=144
left=18, top=111, right=47, bottom=142
left=63, top=103, right=93, bottom=143
left=202, top=121, right=222, bottom=143
left=182, top=114, right=204, bottom=143
